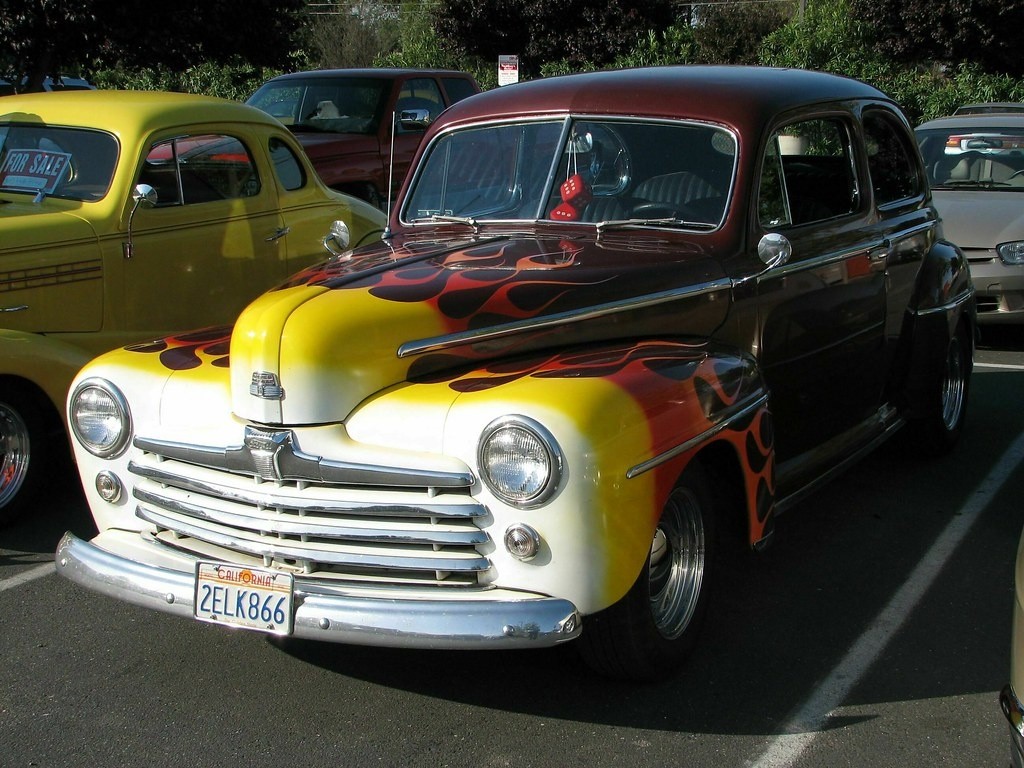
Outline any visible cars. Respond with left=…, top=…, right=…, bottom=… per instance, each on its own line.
left=0, top=89, right=392, bottom=539
left=942, top=102, right=1024, bottom=156
left=48, top=65, right=977, bottom=659
left=905, top=112, right=1024, bottom=342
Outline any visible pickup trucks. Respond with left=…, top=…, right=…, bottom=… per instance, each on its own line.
left=145, top=68, right=593, bottom=214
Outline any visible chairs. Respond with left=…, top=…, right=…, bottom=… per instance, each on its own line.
left=558, top=196, right=633, bottom=223
left=138, top=168, right=225, bottom=204
left=934, top=150, right=986, bottom=180
left=932, top=157, right=970, bottom=180
left=310, top=98, right=352, bottom=119
left=630, top=164, right=850, bottom=225
left=672, top=193, right=728, bottom=225
left=990, top=154, right=1024, bottom=180
